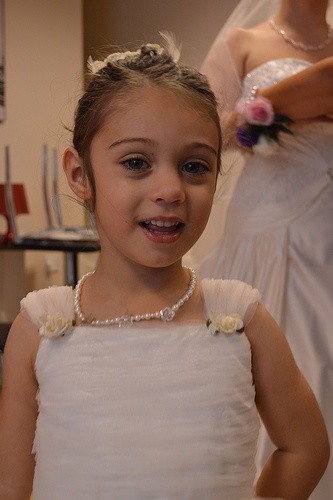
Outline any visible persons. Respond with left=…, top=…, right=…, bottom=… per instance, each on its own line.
left=0, top=45, right=330, bottom=500
left=196, top=0, right=333, bottom=500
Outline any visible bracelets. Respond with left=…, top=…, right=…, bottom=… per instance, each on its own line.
left=237, top=85, right=291, bottom=148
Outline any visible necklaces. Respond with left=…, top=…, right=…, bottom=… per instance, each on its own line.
left=75, top=265, right=196, bottom=325
left=268, top=17, right=331, bottom=50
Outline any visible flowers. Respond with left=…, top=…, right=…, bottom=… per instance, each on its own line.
left=37, top=312, right=76, bottom=338
left=206, top=310, right=246, bottom=336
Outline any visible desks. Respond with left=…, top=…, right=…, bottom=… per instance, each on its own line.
left=1, top=233, right=101, bottom=288
